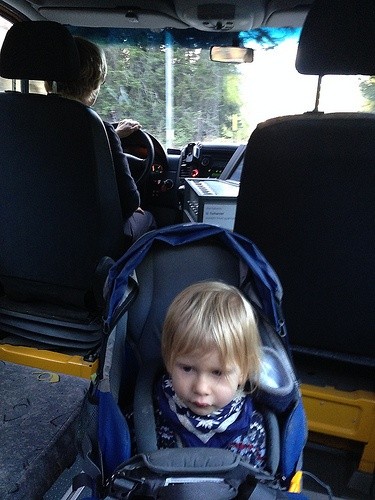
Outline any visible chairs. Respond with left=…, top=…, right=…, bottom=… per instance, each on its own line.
left=233, top=0, right=375, bottom=397
left=0, top=20, right=128, bottom=362
left=0, top=360, right=93, bottom=500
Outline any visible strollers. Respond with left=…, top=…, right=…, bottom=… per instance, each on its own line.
left=58, top=222, right=340, bottom=500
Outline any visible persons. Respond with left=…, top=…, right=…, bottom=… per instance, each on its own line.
left=125, top=281, right=267, bottom=472
left=45, top=37, right=158, bottom=241
